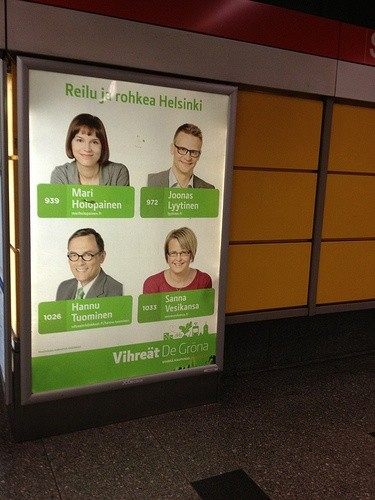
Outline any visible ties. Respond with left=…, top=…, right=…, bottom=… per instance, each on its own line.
left=75, top=287, right=85, bottom=299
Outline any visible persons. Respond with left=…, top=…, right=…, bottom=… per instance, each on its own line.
left=143, top=225, right=212, bottom=294
left=148, top=123, right=215, bottom=190
left=50, top=112, right=129, bottom=186
left=55, top=227, right=124, bottom=301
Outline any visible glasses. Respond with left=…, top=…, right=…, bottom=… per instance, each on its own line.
left=67, top=252, right=99, bottom=261
left=168, top=250, right=190, bottom=257
left=174, top=144, right=200, bottom=158
